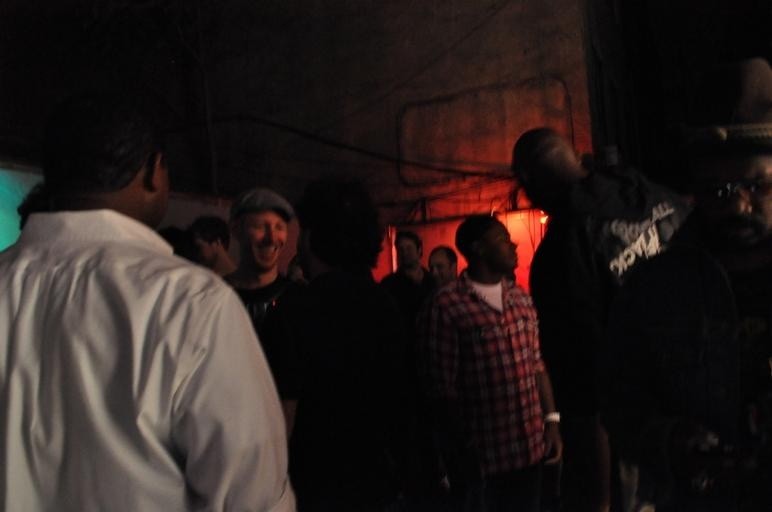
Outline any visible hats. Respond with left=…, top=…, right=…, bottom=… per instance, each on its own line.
left=673, top=49, right=772, bottom=160
left=228, top=185, right=300, bottom=223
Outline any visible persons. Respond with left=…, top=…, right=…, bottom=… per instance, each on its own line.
left=269, top=160, right=430, bottom=510
left=701, top=141, right=771, bottom=290
left=19, top=182, right=46, bottom=230
left=380, top=231, right=431, bottom=314
left=425, top=245, right=457, bottom=287
left=162, top=190, right=299, bottom=320
left=426, top=212, right=565, bottom=509
left=511, top=125, right=771, bottom=511
left=0, top=90, right=301, bottom=511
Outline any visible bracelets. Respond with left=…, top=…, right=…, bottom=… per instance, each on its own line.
left=543, top=412, right=561, bottom=423
